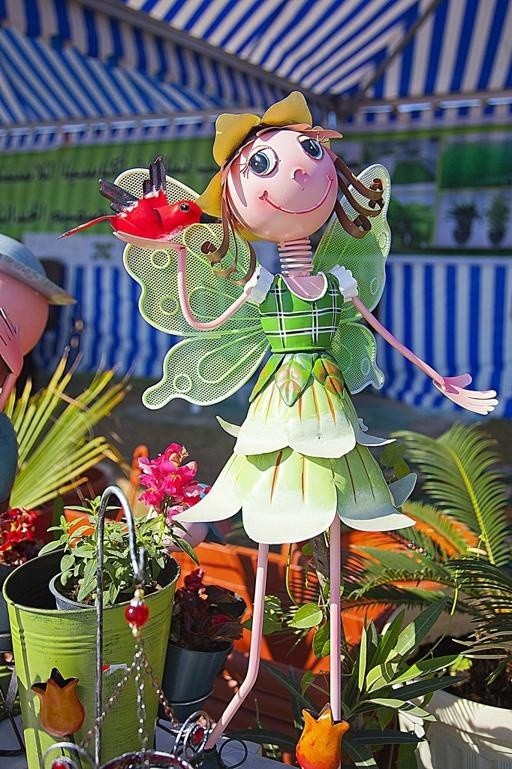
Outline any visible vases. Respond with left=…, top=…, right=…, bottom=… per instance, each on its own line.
left=155, top=642, right=237, bottom=706
left=153, top=681, right=220, bottom=729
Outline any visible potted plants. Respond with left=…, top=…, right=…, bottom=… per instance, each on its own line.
left=267, top=408, right=512, bottom=769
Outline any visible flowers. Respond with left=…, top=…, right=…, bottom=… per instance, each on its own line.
left=0, top=507, right=42, bottom=567
left=138, top=442, right=211, bottom=516
